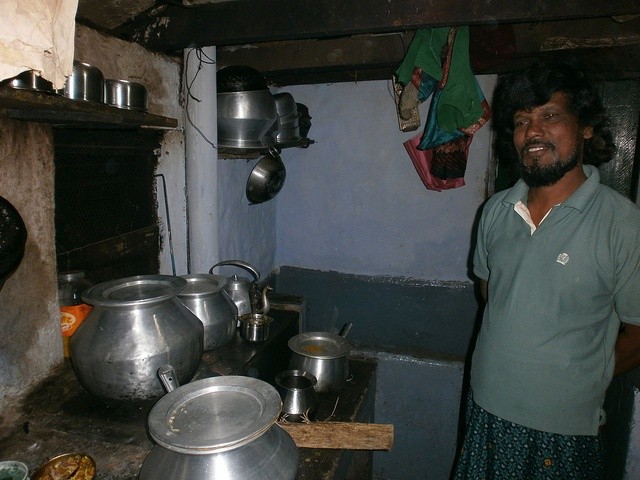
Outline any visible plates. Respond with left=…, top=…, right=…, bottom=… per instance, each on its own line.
left=30, top=452, right=97, bottom=480
left=0, top=459, right=30, bottom=480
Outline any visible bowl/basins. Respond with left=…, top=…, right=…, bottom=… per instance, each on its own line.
left=67, top=272, right=205, bottom=412
left=217, top=90, right=279, bottom=150
left=135, top=372, right=300, bottom=480
left=182, top=274, right=239, bottom=354
left=271, top=93, right=302, bottom=144
left=106, top=80, right=149, bottom=110
left=66, top=60, right=107, bottom=105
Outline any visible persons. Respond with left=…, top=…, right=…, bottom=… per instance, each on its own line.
left=455, top=63, right=640, bottom=479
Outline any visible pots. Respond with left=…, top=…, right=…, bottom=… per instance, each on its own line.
left=246, top=145, right=286, bottom=207
left=286, top=332, right=354, bottom=393
left=217, top=62, right=270, bottom=94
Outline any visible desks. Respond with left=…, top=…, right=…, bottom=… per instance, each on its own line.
left=0, top=294, right=378, bottom=480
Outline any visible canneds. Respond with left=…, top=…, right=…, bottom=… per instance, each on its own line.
left=57, top=270, right=94, bottom=363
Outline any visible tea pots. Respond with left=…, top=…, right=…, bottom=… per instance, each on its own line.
left=207, top=259, right=274, bottom=317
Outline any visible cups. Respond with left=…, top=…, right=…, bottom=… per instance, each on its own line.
left=239, top=313, right=269, bottom=343
left=275, top=368, right=318, bottom=423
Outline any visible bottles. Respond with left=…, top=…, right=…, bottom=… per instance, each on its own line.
left=57, top=271, right=82, bottom=367
